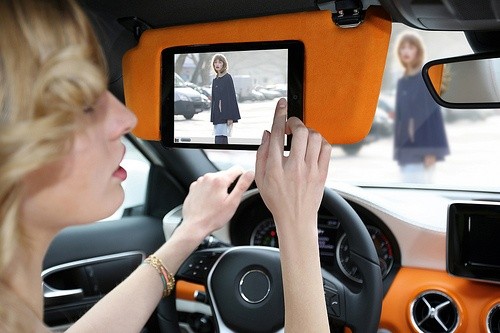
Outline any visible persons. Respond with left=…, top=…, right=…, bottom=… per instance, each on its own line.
left=391, top=32, right=452, bottom=184
left=210, top=54, right=241, bottom=144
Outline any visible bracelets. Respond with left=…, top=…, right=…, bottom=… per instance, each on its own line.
left=0, top=0, right=334, bottom=333
left=143, top=254, right=175, bottom=298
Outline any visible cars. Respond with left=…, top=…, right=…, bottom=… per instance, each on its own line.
left=372, top=95, right=499, bottom=135
left=175, top=73, right=202, bottom=120
left=331, top=105, right=388, bottom=158
left=185, top=83, right=286, bottom=114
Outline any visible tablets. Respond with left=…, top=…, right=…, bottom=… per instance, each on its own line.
left=159, top=40, right=306, bottom=151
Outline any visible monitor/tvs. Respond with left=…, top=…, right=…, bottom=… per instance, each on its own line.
left=446, top=203, right=500, bottom=283
